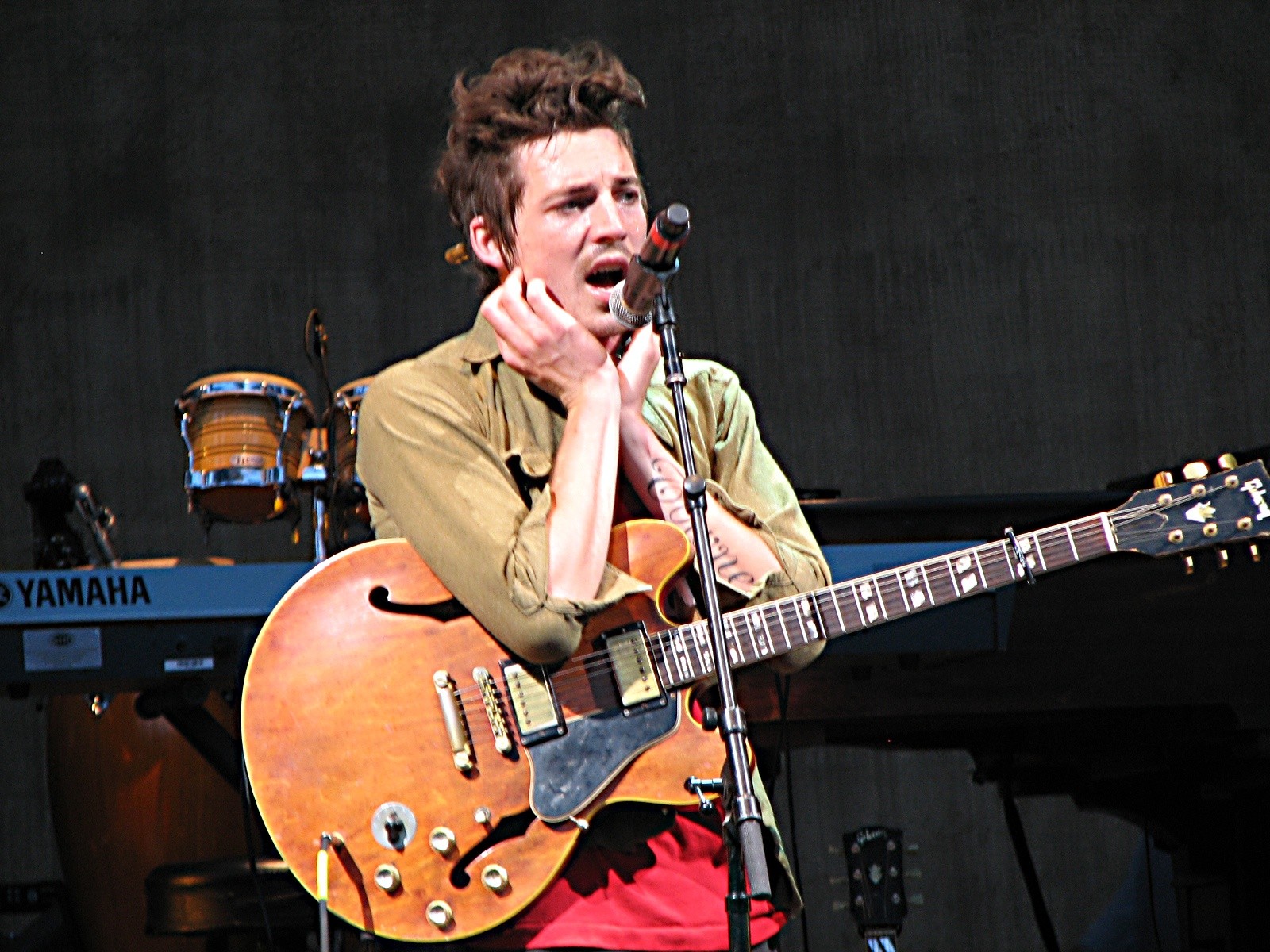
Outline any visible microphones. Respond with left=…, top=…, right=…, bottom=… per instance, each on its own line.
left=609, top=202, right=691, bottom=329
left=313, top=309, right=328, bottom=356
left=72, top=484, right=115, bottom=530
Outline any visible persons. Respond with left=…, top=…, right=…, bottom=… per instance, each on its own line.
left=353, top=38, right=834, bottom=952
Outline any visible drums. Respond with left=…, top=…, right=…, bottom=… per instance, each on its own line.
left=182, top=370, right=381, bottom=519
left=51, top=555, right=239, bottom=952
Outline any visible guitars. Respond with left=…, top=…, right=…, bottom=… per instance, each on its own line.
left=240, top=452, right=1270, bottom=947
